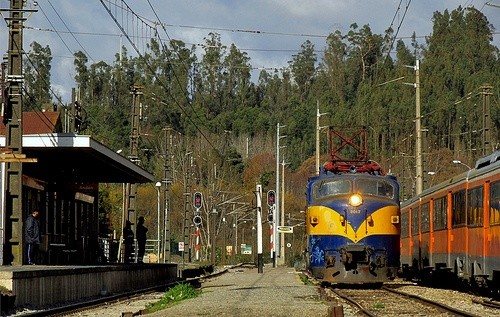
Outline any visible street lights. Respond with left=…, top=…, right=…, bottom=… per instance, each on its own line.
left=155, top=182, right=161, bottom=262
left=428, top=171, right=438, bottom=183
left=452, top=159, right=471, bottom=170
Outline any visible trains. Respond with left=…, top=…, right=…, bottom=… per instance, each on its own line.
left=400, top=150, right=500, bottom=300
left=304, top=159, right=402, bottom=288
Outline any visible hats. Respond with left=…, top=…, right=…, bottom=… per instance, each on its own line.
left=138, top=216, right=145, bottom=221
left=126, top=221, right=134, bottom=226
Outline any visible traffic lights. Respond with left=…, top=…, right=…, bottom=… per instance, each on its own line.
left=268, top=190, right=275, bottom=207
left=194, top=192, right=202, bottom=208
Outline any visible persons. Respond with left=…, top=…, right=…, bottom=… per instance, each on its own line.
left=24, top=207, right=40, bottom=265
left=136, top=216, right=148, bottom=263
left=122, top=219, right=134, bottom=263
